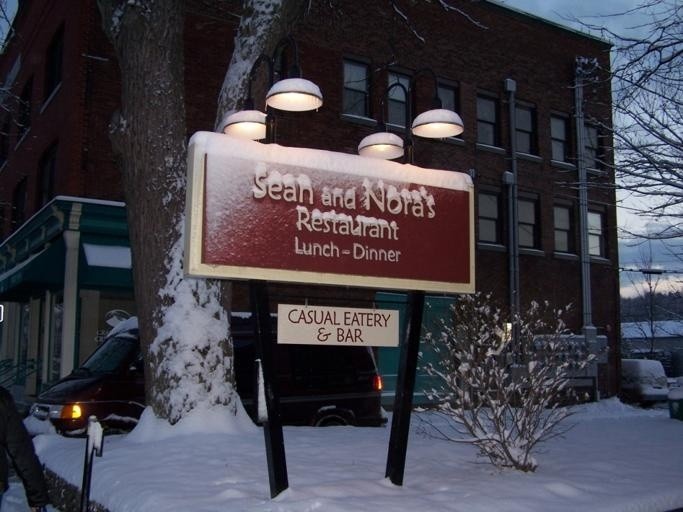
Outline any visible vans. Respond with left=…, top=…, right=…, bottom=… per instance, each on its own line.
left=31, top=308, right=390, bottom=439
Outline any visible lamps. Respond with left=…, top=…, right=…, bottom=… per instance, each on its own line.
left=214, top=29, right=468, bottom=164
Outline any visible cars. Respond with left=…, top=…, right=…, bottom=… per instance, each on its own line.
left=621, top=358, right=683, bottom=421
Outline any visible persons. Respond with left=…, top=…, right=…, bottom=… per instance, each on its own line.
left=0, top=385, right=51, bottom=512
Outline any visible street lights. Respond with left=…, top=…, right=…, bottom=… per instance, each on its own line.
left=215, top=75, right=325, bottom=502
left=357, top=105, right=467, bottom=489
